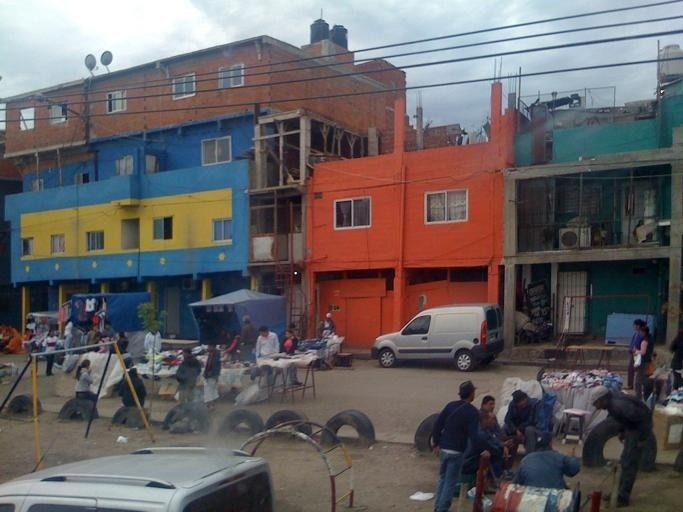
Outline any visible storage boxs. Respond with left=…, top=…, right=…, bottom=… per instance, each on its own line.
left=652, top=402, right=682, bottom=452
left=334, top=351, right=353, bottom=367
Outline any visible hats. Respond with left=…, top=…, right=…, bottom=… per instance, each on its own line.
left=457, top=381, right=477, bottom=395
left=590, top=385, right=609, bottom=405
left=512, top=390, right=528, bottom=401
left=536, top=432, right=552, bottom=447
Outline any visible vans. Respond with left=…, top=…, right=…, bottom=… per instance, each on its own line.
left=0, top=448, right=277, bottom=512
left=371, top=304, right=504, bottom=372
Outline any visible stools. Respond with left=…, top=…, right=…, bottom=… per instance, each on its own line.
left=561, top=408, right=590, bottom=447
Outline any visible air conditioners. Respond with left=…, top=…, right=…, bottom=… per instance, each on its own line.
left=559, top=228, right=591, bottom=250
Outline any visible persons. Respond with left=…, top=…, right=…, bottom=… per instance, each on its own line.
left=119, top=367, right=145, bottom=408
left=199, top=343, right=221, bottom=411
left=316, top=309, right=336, bottom=368
left=475, top=394, right=504, bottom=435
left=176, top=346, right=200, bottom=405
left=45, top=313, right=129, bottom=375
left=625, top=319, right=645, bottom=388
left=143, top=326, right=161, bottom=379
left=248, top=325, right=279, bottom=381
left=589, top=382, right=653, bottom=505
left=631, top=323, right=653, bottom=400
left=279, top=324, right=302, bottom=385
left=239, top=314, right=256, bottom=359
left=512, top=430, right=578, bottom=488
left=221, top=329, right=240, bottom=354
left=670, top=323, right=682, bottom=388
left=74, top=360, right=99, bottom=400
left=460, top=412, right=506, bottom=474
left=428, top=378, right=488, bottom=510
left=500, top=389, right=550, bottom=456
